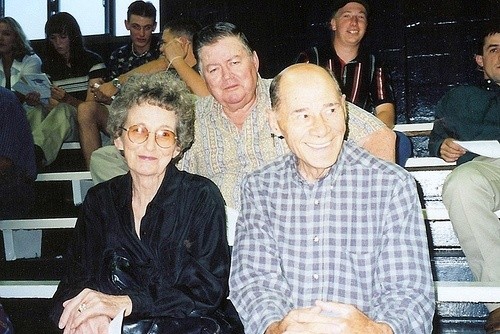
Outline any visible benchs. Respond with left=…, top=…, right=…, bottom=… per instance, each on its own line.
left=0, top=123, right=500, bottom=334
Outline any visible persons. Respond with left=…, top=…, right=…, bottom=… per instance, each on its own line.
left=227, top=62, right=436, bottom=334
left=0, top=0, right=413, bottom=219
left=426, top=22, right=500, bottom=334
left=49, top=70, right=228, bottom=334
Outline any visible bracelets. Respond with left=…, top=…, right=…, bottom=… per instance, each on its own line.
left=166, top=57, right=181, bottom=74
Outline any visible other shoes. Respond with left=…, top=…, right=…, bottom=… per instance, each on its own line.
left=484, top=308, right=500, bottom=333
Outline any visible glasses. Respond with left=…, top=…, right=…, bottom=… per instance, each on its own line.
left=121, top=124, right=179, bottom=148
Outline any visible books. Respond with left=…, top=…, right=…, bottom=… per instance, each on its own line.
left=13, top=72, right=53, bottom=100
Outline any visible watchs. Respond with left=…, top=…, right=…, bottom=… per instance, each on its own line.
left=112, top=77, right=122, bottom=90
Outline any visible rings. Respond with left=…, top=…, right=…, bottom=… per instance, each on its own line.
left=77, top=303, right=88, bottom=313
